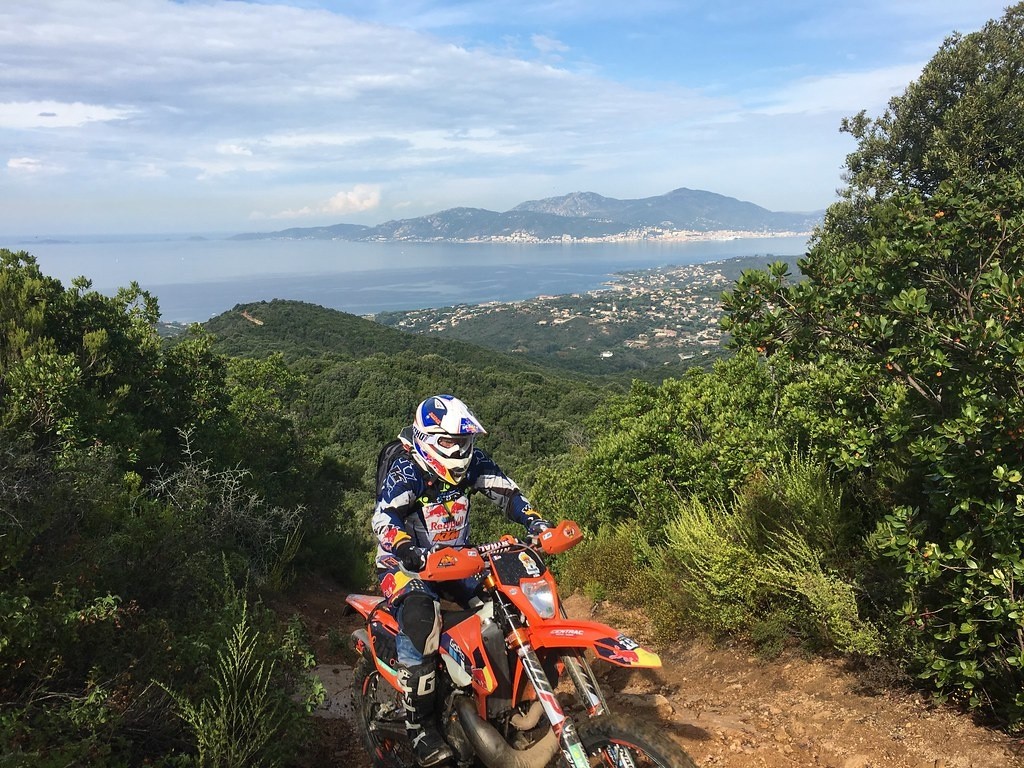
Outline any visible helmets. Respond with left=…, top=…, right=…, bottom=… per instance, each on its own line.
left=412, top=394, right=489, bottom=485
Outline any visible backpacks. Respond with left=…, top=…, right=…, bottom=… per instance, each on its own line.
left=376, top=439, right=430, bottom=502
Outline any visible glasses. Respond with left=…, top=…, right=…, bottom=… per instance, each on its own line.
left=432, top=434, right=473, bottom=456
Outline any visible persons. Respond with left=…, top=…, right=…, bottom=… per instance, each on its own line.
left=371, top=394, right=554, bottom=768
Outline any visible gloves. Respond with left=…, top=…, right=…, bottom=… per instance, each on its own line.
left=529, top=521, right=556, bottom=536
left=396, top=542, right=422, bottom=573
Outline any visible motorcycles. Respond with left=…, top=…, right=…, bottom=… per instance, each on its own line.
left=341, top=518, right=702, bottom=768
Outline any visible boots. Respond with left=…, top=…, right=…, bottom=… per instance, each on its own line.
left=397, top=662, right=454, bottom=768
